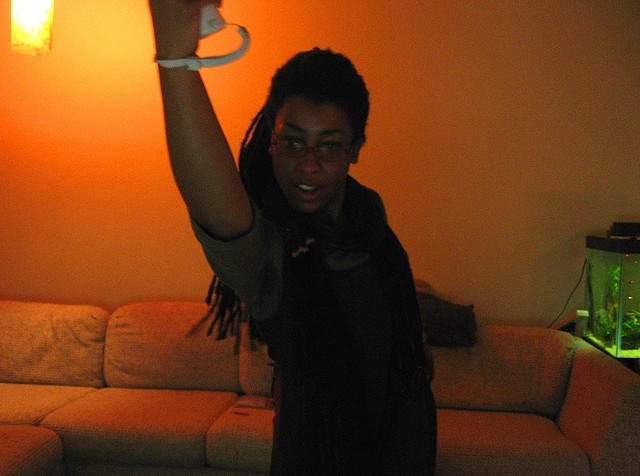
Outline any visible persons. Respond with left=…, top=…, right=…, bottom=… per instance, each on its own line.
left=147, top=0, right=437, bottom=476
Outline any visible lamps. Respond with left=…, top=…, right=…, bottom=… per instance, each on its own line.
left=10, top=0, right=56, bottom=56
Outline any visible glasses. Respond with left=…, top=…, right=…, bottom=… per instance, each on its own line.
left=276, top=137, right=346, bottom=163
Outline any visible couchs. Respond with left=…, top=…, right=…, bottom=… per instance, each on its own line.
left=0, top=296, right=639, bottom=476
